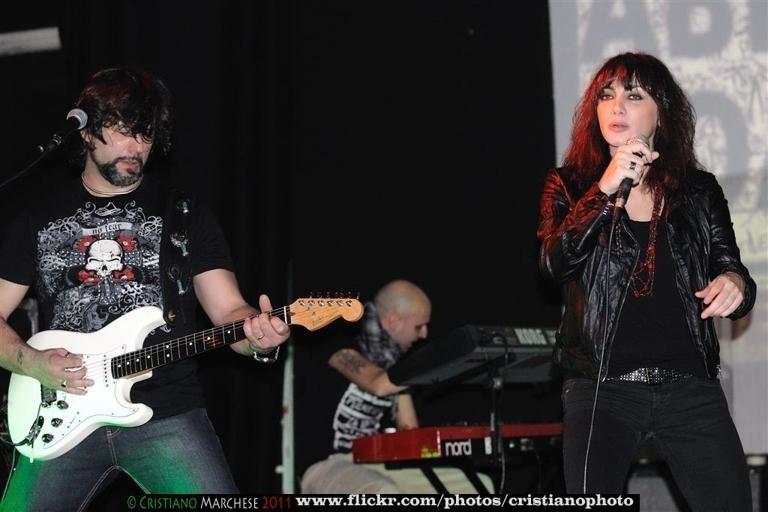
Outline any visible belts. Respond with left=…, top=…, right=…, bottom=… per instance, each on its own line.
left=601, top=367, right=729, bottom=387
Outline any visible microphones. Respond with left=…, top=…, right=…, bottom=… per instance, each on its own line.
left=39, top=107, right=89, bottom=154
left=610, top=131, right=652, bottom=225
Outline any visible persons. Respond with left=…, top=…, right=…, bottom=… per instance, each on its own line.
left=536, top=50, right=757, bottom=512
left=294, top=278, right=497, bottom=494
left=0, top=64, right=293, bottom=512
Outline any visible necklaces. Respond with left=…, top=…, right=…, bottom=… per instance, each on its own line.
left=79, top=170, right=143, bottom=196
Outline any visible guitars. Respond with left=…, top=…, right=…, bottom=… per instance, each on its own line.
left=6, top=290, right=364, bottom=461
left=352, top=424, right=563, bottom=469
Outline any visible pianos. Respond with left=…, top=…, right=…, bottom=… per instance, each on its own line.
left=386, top=324, right=560, bottom=386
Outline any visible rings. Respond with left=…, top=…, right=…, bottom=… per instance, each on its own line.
left=61, top=380, right=67, bottom=388
left=630, top=161, right=637, bottom=169
left=256, top=334, right=265, bottom=341
left=65, top=351, right=72, bottom=356
